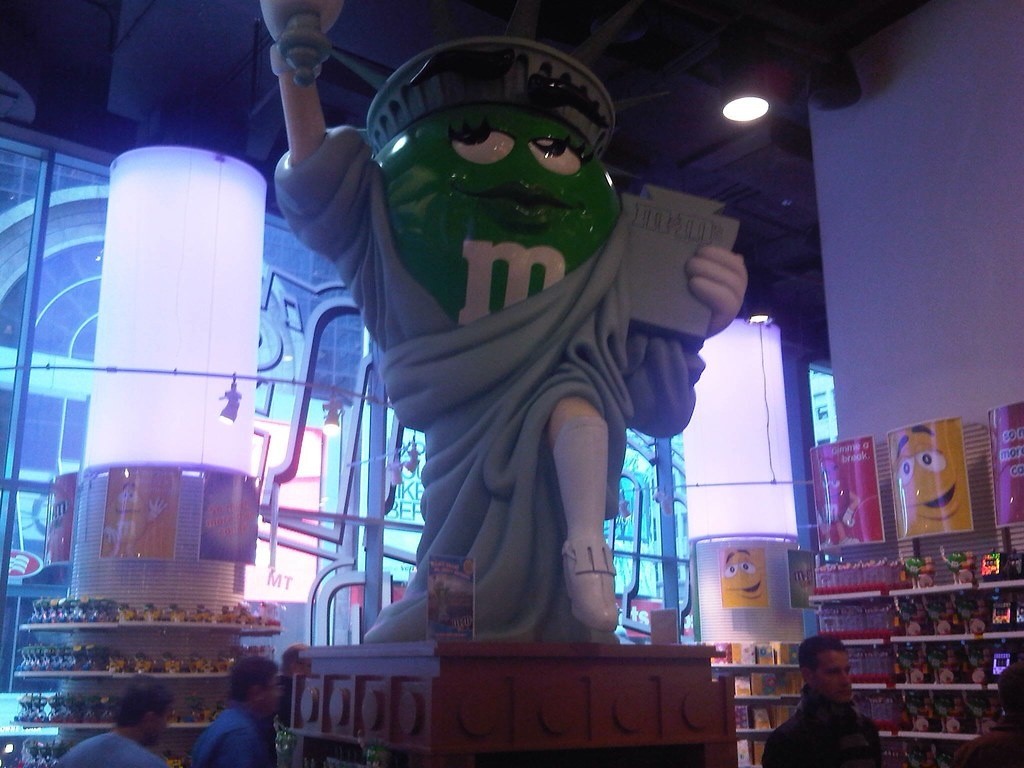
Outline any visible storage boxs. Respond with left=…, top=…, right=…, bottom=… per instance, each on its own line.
left=705, top=640, right=805, bottom=768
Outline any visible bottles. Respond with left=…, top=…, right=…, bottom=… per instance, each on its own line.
left=813, top=558, right=905, bottom=768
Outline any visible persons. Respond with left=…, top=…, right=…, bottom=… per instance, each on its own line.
left=259, top=0, right=750, bottom=637
left=191, top=655, right=282, bottom=768
left=952, top=661, right=1024, bottom=768
left=274, top=644, right=312, bottom=727
left=761, top=634, right=883, bottom=768
left=54, top=673, right=173, bottom=768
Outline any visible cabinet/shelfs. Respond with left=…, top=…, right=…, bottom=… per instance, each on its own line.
left=711, top=663, right=801, bottom=733
left=808, top=579, right=1024, bottom=741
left=9, top=620, right=288, bottom=728
